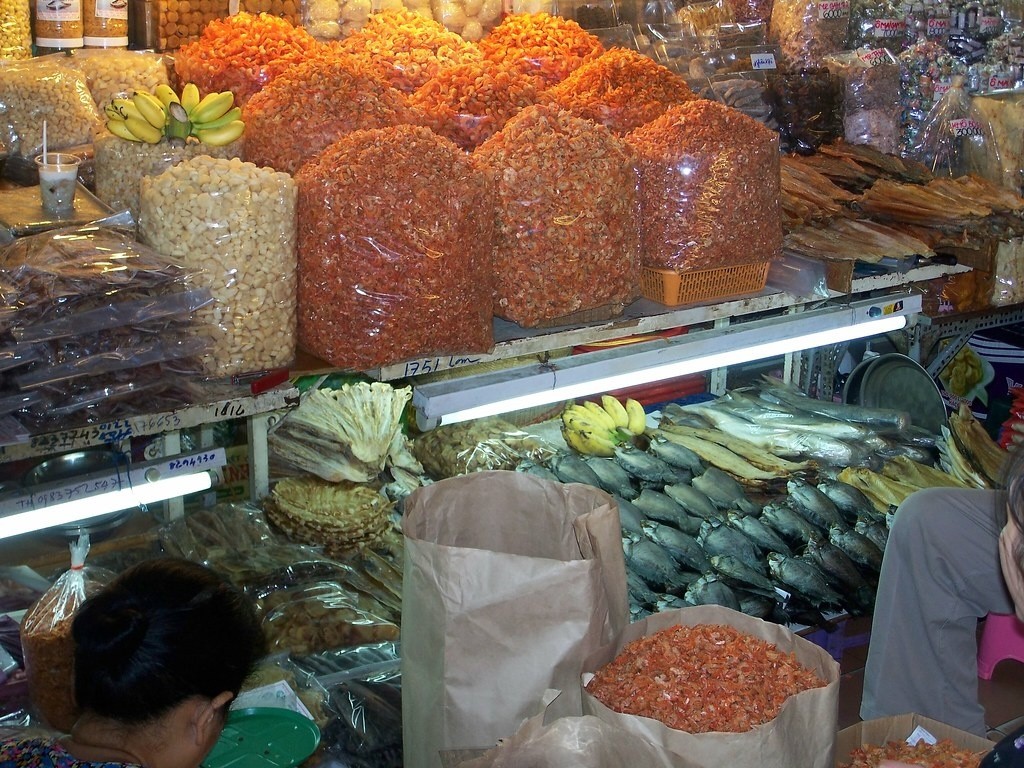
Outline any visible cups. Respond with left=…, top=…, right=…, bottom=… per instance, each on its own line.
left=33, top=153, right=82, bottom=218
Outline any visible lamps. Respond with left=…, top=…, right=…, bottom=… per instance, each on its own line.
left=0, top=471, right=222, bottom=539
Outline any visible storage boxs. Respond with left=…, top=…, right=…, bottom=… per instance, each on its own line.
left=150, top=436, right=249, bottom=515
left=834, top=711, right=997, bottom=768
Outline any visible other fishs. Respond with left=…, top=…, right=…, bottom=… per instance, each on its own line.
left=517, top=435, right=891, bottom=634
left=779, top=139, right=1022, bottom=260
left=657, top=374, right=936, bottom=488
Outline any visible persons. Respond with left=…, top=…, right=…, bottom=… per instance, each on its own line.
left=0, top=557, right=268, bottom=768
left=860, top=440, right=1024, bottom=768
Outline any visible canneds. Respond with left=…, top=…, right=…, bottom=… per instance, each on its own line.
left=33, top=0, right=128, bottom=58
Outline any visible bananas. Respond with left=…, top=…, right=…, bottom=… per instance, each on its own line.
left=560, top=394, right=646, bottom=458
left=104, top=81, right=246, bottom=147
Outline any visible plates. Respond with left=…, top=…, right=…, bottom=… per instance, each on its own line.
left=841, top=352, right=949, bottom=436
